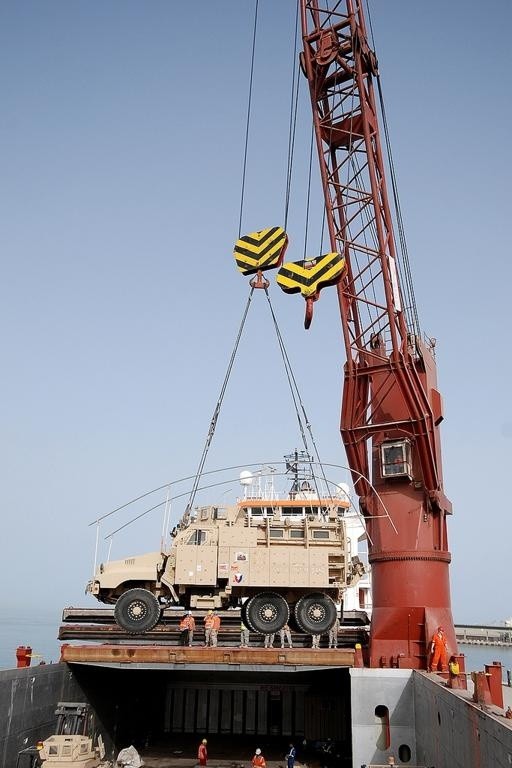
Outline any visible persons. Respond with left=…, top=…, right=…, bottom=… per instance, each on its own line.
left=321, top=736, right=336, bottom=768
left=250, top=747, right=267, bottom=768
left=197, top=738, right=209, bottom=768
left=284, top=743, right=297, bottom=768
left=179, top=609, right=195, bottom=648
left=393, top=453, right=404, bottom=473
left=430, top=627, right=449, bottom=672
left=202, top=610, right=221, bottom=648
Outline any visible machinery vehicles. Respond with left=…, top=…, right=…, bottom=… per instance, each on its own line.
left=86, top=494, right=371, bottom=631
left=16, top=698, right=120, bottom=768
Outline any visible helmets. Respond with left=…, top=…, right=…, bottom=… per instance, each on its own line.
left=201, top=739, right=207, bottom=744
left=208, top=609, right=213, bottom=615
left=438, top=627, right=444, bottom=631
left=256, top=748, right=261, bottom=754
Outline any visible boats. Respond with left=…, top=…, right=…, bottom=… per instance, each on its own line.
left=0, top=3, right=508, bottom=768
